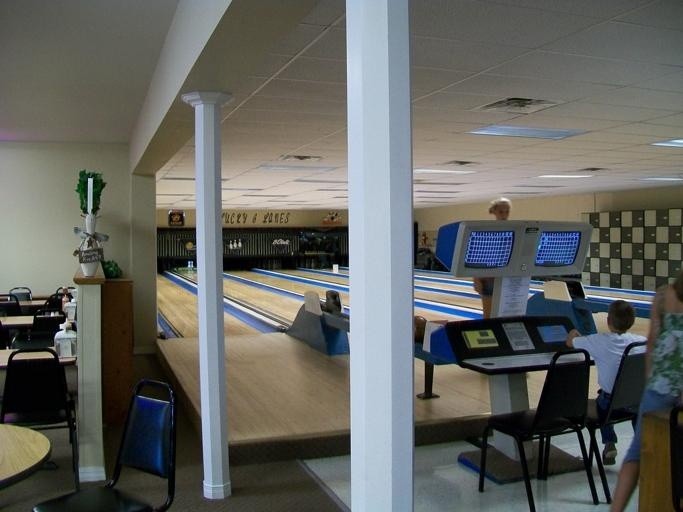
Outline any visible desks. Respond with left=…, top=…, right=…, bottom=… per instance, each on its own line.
left=1, top=422, right=51, bottom=490
left=0, top=349, right=76, bottom=368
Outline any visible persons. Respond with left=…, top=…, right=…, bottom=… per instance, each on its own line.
left=473, top=195, right=511, bottom=318
left=606, top=268, right=682, bottom=511
left=565, top=300, right=647, bottom=466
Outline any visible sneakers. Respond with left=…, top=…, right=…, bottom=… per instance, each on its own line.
left=602, top=444, right=618, bottom=467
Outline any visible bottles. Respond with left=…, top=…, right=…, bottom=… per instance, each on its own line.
left=62, top=294, right=69, bottom=311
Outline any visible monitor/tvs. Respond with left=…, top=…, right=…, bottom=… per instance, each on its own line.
left=532, top=221, right=594, bottom=276
left=435, top=221, right=542, bottom=278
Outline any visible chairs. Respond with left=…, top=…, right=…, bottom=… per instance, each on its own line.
left=0, top=348, right=84, bottom=490
left=543, top=340, right=647, bottom=505
left=479, top=348, right=599, bottom=512
left=33, top=378, right=175, bottom=512
left=0, top=286, right=76, bottom=350
